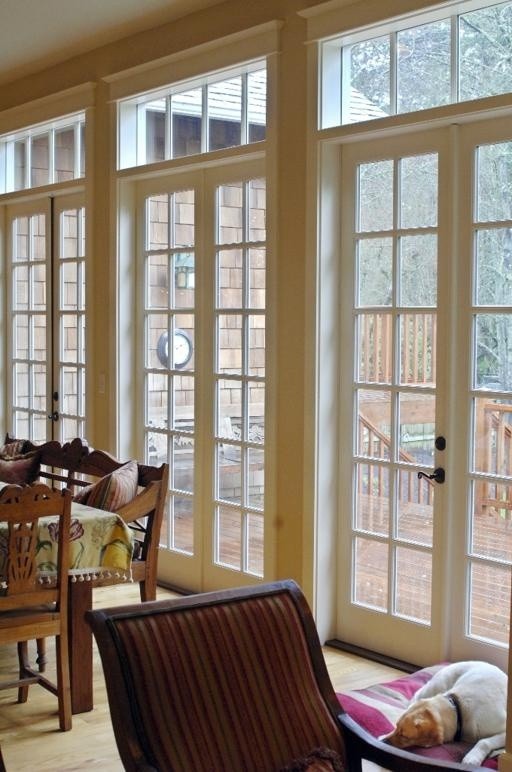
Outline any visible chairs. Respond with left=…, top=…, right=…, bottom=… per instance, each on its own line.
left=1, top=432, right=176, bottom=737
left=82, top=572, right=496, bottom=772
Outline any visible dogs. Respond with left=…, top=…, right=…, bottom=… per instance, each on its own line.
left=379, top=659, right=508, bottom=768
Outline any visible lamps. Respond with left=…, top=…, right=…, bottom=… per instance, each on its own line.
left=176, top=244, right=196, bottom=290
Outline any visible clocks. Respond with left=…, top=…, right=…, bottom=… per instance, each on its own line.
left=157, top=327, right=194, bottom=371
